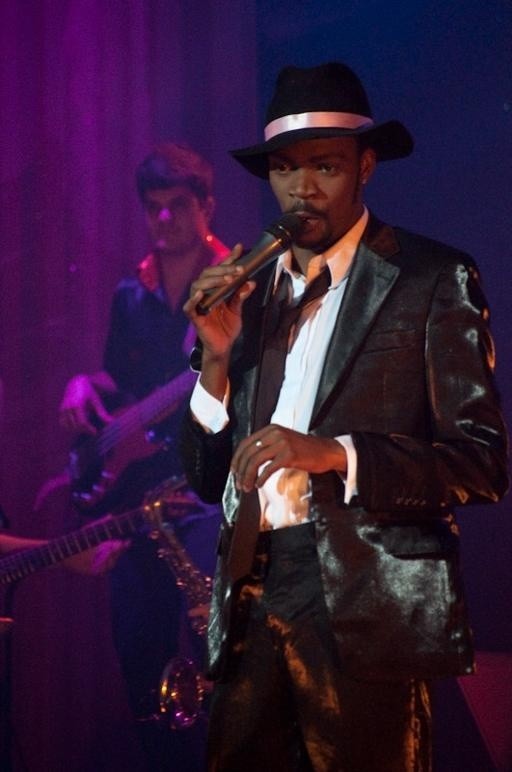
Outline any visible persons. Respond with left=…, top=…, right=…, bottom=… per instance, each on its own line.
left=60, top=142, right=240, bottom=726
left=0, top=513, right=132, bottom=579
left=184, top=66, right=512, bottom=772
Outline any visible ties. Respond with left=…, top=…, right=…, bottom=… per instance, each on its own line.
left=226, top=266, right=331, bottom=586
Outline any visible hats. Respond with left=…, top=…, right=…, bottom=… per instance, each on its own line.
left=228, top=63, right=413, bottom=180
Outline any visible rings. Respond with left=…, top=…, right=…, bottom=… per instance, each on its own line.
left=254, top=438, right=268, bottom=450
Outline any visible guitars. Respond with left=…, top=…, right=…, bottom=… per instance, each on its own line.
left=64, top=370, right=198, bottom=505
left=0, top=493, right=208, bottom=586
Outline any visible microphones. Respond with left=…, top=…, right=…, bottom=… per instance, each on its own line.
left=194, top=213, right=304, bottom=315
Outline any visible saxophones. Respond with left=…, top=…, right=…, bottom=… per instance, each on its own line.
left=143, top=479, right=217, bottom=727
